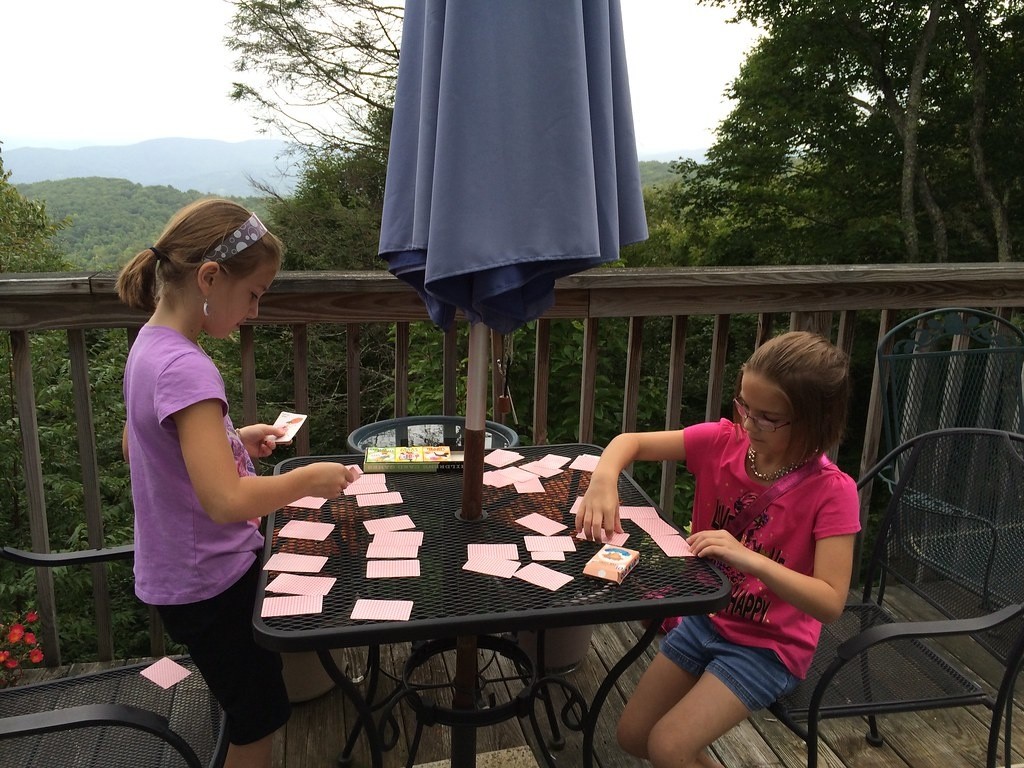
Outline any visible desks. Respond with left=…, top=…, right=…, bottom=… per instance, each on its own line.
left=252, top=443, right=732, bottom=768
left=347, top=414, right=519, bottom=457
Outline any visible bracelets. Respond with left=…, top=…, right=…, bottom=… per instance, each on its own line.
left=232, top=427, right=241, bottom=445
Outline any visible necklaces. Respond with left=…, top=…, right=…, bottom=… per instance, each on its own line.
left=748, top=445, right=820, bottom=484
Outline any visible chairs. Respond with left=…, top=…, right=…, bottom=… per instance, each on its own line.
left=0, top=543, right=232, bottom=768
left=877, top=307, right=1024, bottom=638
left=764, top=428, right=1024, bottom=768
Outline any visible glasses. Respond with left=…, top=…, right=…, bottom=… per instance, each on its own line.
left=734, top=396, right=791, bottom=432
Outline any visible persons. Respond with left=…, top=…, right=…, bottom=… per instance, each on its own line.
left=108, top=195, right=359, bottom=766
left=572, top=328, right=868, bottom=766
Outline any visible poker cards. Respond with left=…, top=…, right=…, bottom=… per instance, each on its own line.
left=137, top=410, right=700, bottom=691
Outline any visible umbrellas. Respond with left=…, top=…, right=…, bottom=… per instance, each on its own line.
left=374, top=0, right=653, bottom=526
